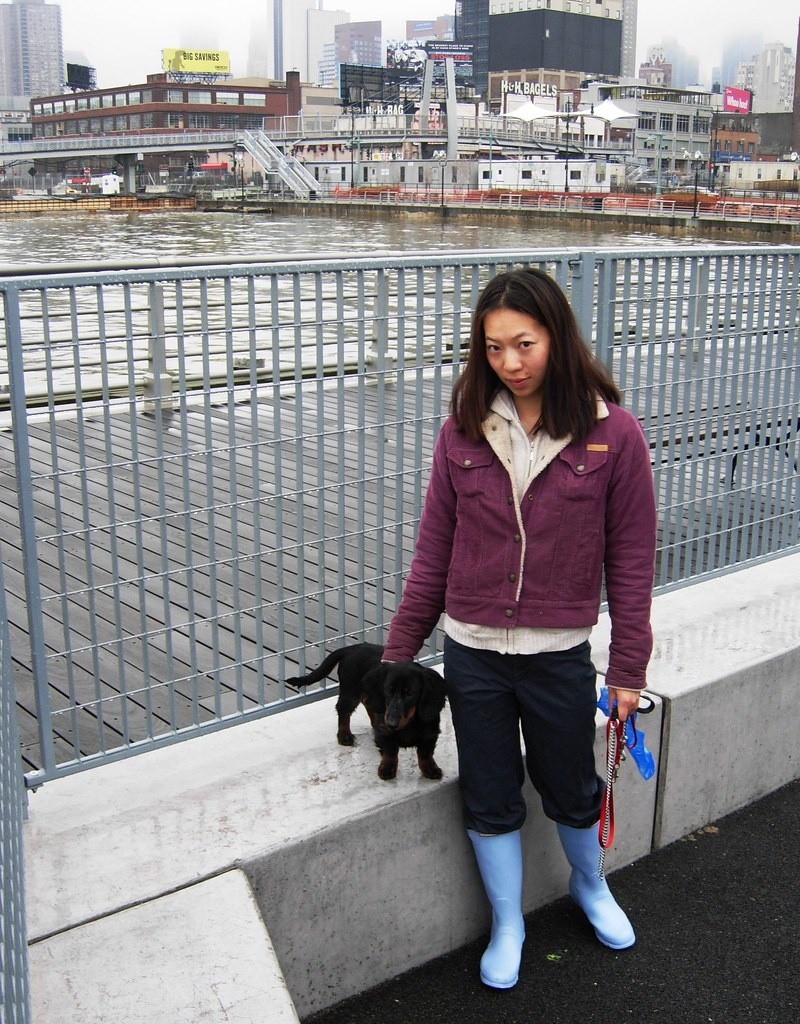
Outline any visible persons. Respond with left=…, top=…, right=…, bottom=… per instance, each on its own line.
left=380, top=266, right=658, bottom=989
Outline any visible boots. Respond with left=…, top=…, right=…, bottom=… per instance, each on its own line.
left=466, top=828, right=526, bottom=988
left=556, top=821, right=636, bottom=949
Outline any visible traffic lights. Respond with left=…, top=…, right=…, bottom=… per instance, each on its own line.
left=111, top=166, right=117, bottom=174
left=188, top=162, right=194, bottom=171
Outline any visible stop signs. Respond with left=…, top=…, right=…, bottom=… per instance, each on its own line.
left=84, top=167, right=90, bottom=176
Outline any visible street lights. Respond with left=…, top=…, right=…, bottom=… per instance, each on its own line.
left=438, top=160, right=447, bottom=208
left=476, top=132, right=493, bottom=189
left=690, top=160, right=702, bottom=219
left=643, top=132, right=665, bottom=199
left=231, top=164, right=247, bottom=202
left=345, top=135, right=361, bottom=188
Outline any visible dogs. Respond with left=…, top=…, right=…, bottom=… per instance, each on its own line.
left=284, top=641, right=448, bottom=781
